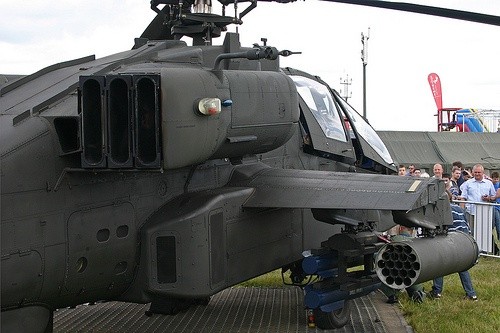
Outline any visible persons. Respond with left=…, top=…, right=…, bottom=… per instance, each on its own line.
left=399, top=162, right=473, bottom=197
left=426, top=189, right=478, bottom=301
left=491, top=171, right=500, bottom=256
left=385, top=224, right=426, bottom=305
left=460, top=163, right=496, bottom=259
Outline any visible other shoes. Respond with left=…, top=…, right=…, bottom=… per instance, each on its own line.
left=428, top=291, right=441, bottom=300
left=463, top=295, right=478, bottom=302
left=386, top=295, right=398, bottom=304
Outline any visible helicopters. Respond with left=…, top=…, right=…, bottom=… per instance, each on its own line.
left=0, top=0, right=500, bottom=333
